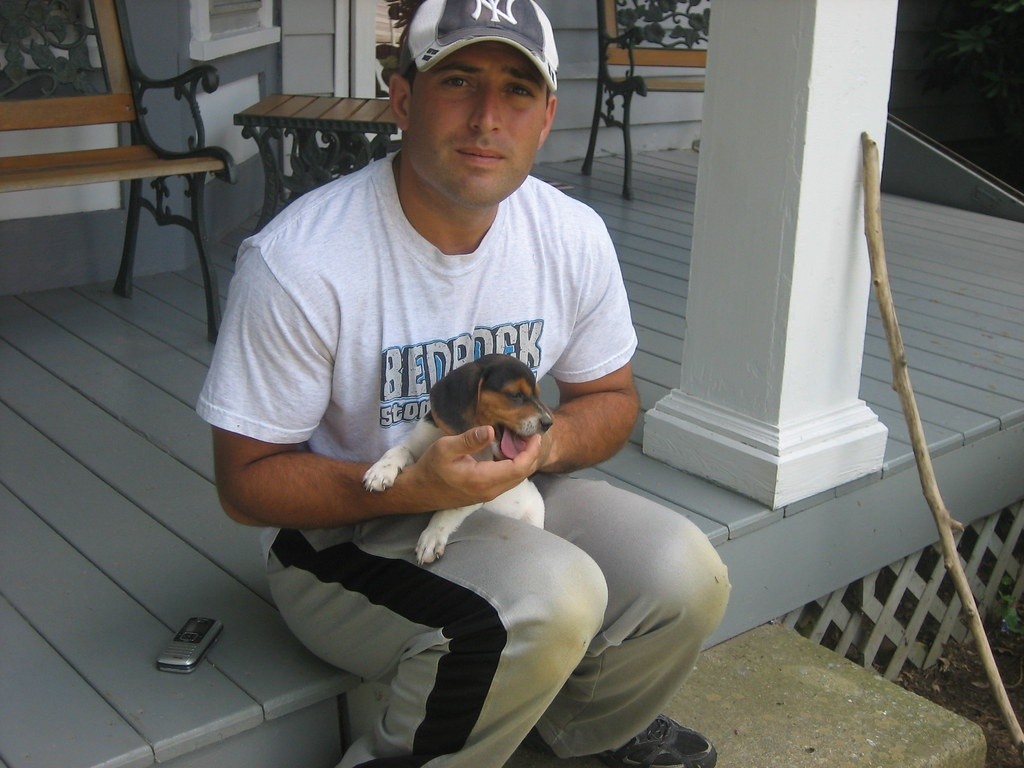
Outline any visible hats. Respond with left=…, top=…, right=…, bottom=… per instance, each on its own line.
left=398, top=0, right=558, bottom=91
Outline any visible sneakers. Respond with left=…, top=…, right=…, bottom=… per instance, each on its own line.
left=523, top=714, right=718, bottom=768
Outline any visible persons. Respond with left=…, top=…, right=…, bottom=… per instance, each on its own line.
left=195, top=0, right=731, bottom=768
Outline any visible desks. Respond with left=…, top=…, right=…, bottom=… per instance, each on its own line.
left=233, top=94, right=400, bottom=235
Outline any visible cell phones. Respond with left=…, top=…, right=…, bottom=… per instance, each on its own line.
left=157, top=616, right=224, bottom=673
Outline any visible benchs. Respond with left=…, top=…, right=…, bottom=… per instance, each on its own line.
left=581, top=0, right=712, bottom=199
left=0, top=0, right=240, bottom=345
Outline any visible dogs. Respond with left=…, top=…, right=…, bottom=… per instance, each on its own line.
left=362, top=355, right=553, bottom=565
